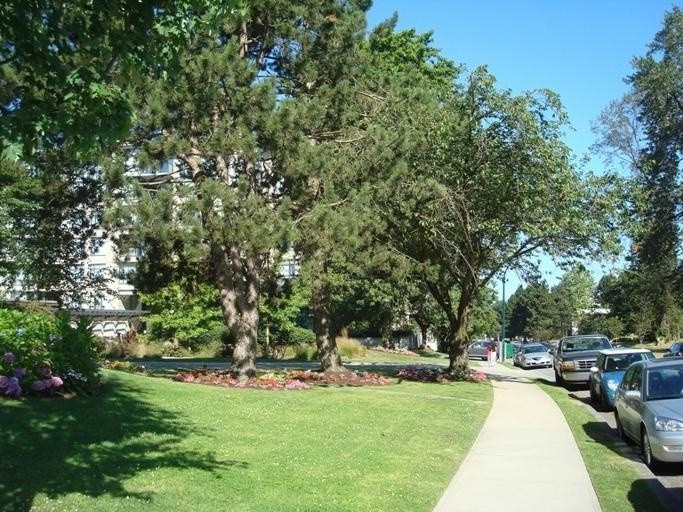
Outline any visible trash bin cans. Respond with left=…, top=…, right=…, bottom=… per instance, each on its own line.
left=502, top=338, right=513, bottom=359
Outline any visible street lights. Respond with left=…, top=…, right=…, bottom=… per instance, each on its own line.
left=502, top=254, right=539, bottom=361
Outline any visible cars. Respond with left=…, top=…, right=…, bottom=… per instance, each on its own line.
left=513, top=343, right=554, bottom=368
left=467, top=340, right=491, bottom=361
left=551, top=335, right=683, bottom=471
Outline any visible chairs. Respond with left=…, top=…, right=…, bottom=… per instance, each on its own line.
left=592, top=342, right=600, bottom=348
left=603, top=358, right=619, bottom=370
left=647, top=372, right=673, bottom=397
left=566, top=343, right=574, bottom=348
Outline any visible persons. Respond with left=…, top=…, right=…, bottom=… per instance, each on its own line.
left=486, top=341, right=497, bottom=367
